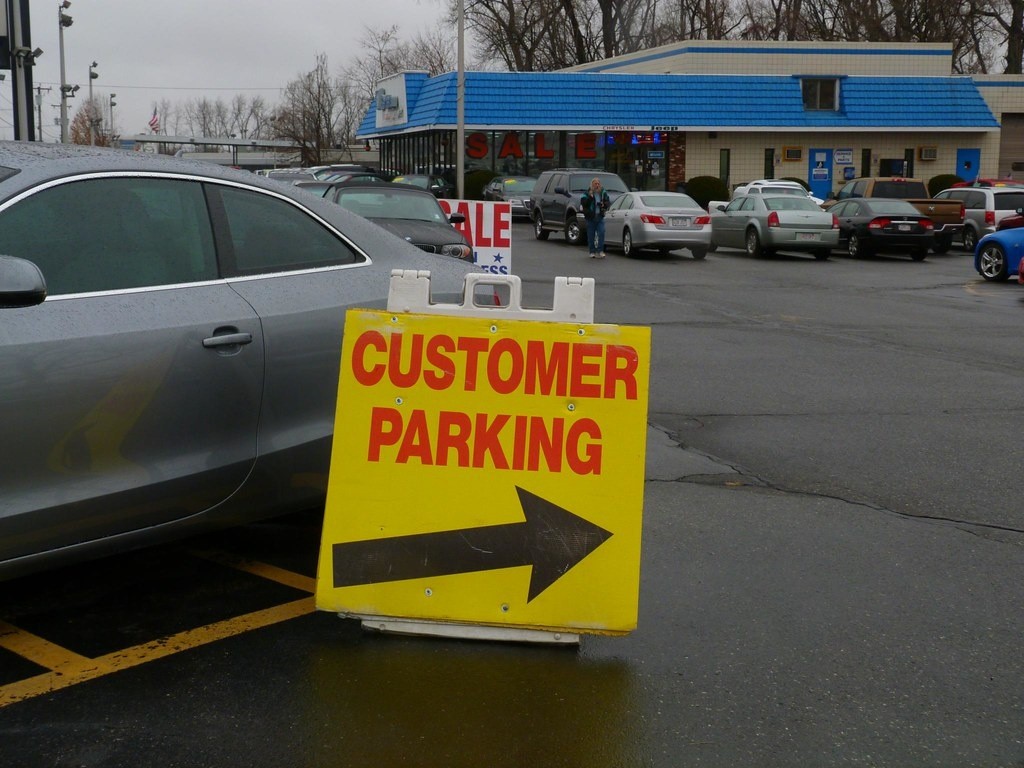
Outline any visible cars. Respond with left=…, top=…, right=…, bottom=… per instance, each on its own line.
left=482, top=175, right=537, bottom=217
left=933, top=179, right=1024, bottom=281
left=255, top=164, right=474, bottom=265
left=595, top=191, right=711, bottom=260
left=825, top=198, right=935, bottom=261
left=710, top=179, right=839, bottom=259
left=1, top=139, right=494, bottom=568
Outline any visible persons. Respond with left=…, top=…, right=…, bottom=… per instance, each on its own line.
left=581, top=178, right=610, bottom=258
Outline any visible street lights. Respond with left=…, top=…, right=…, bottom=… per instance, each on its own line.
left=57, top=1, right=80, bottom=144
left=89, top=61, right=98, bottom=145
left=109, top=93, right=121, bottom=149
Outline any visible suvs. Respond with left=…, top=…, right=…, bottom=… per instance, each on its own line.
left=529, top=167, right=638, bottom=244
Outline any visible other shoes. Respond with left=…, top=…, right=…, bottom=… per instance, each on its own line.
left=600, top=251, right=606, bottom=258
left=589, top=253, right=595, bottom=258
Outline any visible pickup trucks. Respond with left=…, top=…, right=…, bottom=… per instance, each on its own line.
left=821, top=177, right=965, bottom=254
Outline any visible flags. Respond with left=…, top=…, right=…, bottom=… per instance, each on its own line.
left=149, top=109, right=159, bottom=133
